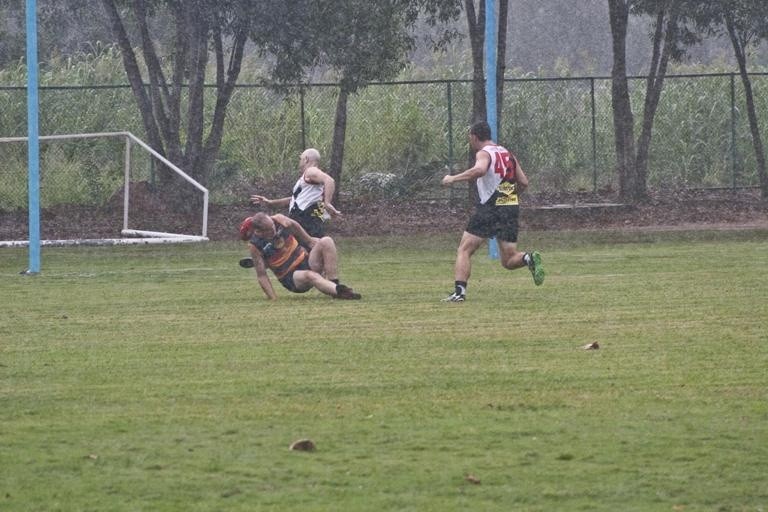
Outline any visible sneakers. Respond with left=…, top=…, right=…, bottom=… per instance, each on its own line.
left=330, top=278, right=362, bottom=299
left=439, top=285, right=466, bottom=303
left=239, top=258, right=255, bottom=268
left=526, top=250, right=546, bottom=287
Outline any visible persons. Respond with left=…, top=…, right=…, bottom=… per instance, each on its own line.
left=239, top=148, right=344, bottom=268
left=440, top=120, right=544, bottom=303
left=245, top=212, right=363, bottom=301
